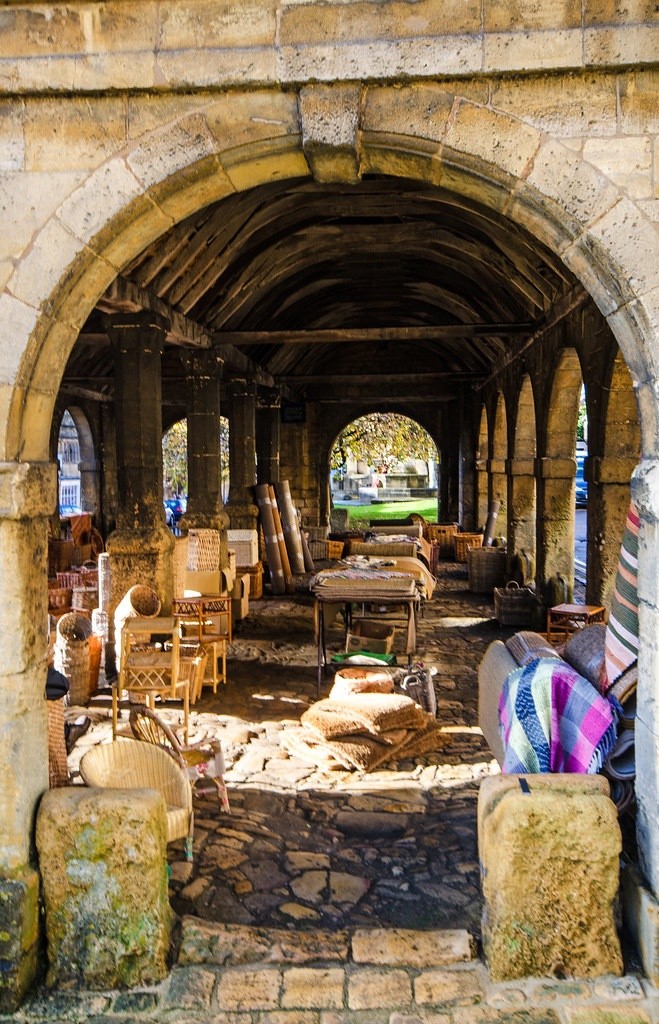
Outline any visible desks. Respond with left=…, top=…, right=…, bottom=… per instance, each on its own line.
left=313, top=596, right=422, bottom=701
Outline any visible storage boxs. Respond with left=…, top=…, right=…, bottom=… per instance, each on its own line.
left=183, top=530, right=263, bottom=645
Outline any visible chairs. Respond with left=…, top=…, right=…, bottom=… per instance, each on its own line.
left=79, top=740, right=194, bottom=862
left=129, top=706, right=231, bottom=813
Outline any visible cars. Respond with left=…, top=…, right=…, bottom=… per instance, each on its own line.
left=59, top=504, right=82, bottom=518
left=162, top=498, right=187, bottom=527
left=574, top=450, right=588, bottom=510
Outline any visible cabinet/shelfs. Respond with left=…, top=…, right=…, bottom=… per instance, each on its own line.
left=113, top=596, right=232, bottom=748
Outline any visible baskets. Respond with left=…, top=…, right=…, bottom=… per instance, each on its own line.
left=466, top=542, right=497, bottom=593
left=48, top=537, right=98, bottom=613
left=113, top=582, right=161, bottom=691
left=428, top=521, right=458, bottom=551
left=400, top=665, right=437, bottom=717
left=53, top=612, right=93, bottom=708
left=494, top=581, right=536, bottom=626
left=454, top=532, right=484, bottom=563
